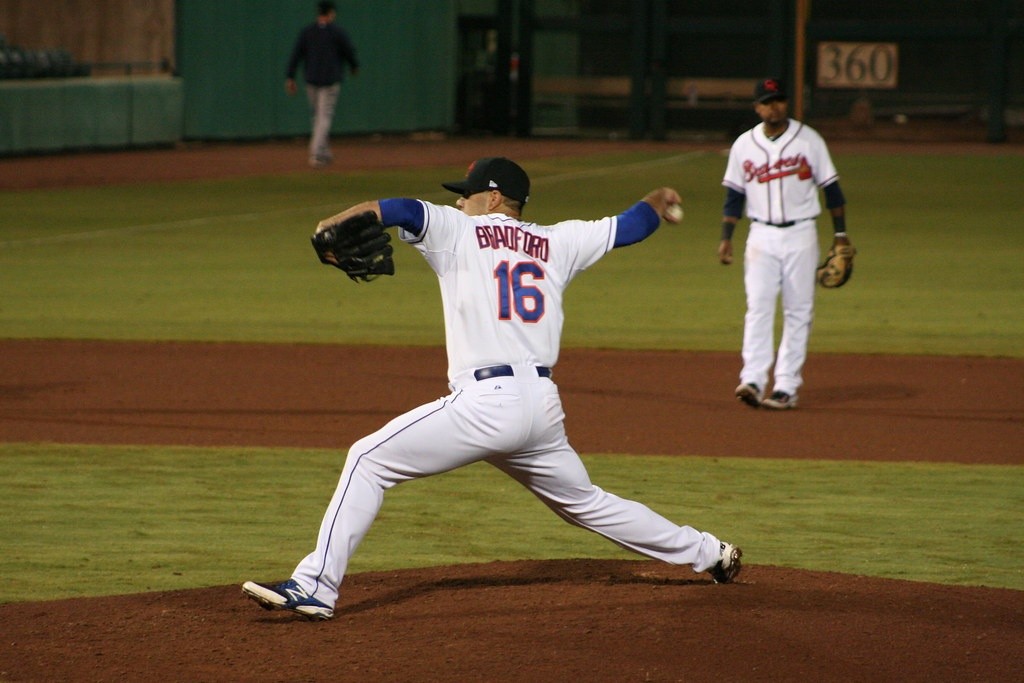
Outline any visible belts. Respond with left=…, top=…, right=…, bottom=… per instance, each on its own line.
left=755, top=217, right=811, bottom=229
left=474, top=365, right=551, bottom=381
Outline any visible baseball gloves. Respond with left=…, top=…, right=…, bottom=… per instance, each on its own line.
left=817, top=244, right=861, bottom=291
left=312, top=209, right=396, bottom=280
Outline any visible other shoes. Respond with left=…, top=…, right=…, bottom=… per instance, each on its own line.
left=310, top=155, right=331, bottom=169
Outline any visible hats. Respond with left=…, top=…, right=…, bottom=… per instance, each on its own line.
left=754, top=78, right=787, bottom=102
left=441, top=156, right=531, bottom=204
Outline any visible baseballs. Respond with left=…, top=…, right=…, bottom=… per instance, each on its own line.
left=667, top=205, right=683, bottom=221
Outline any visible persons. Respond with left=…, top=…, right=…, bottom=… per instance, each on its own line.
left=242, top=156, right=743, bottom=619
left=287, top=0, right=360, bottom=165
left=718, top=78, right=856, bottom=408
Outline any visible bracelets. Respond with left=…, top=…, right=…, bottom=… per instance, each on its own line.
left=832, top=215, right=847, bottom=237
left=721, top=222, right=735, bottom=239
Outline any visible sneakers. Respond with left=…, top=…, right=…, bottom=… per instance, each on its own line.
left=241, top=579, right=334, bottom=621
left=735, top=384, right=763, bottom=407
left=708, top=541, right=742, bottom=583
left=761, top=390, right=799, bottom=409
